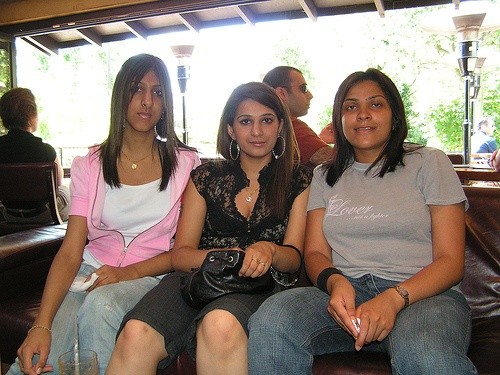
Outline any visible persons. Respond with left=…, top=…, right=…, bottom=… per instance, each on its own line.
left=263, top=64, right=500, bottom=176
left=106, top=79, right=313, bottom=375
left=248, top=67, right=478, bottom=375
left=0, top=87, right=71, bottom=226
left=6, top=53, right=201, bottom=375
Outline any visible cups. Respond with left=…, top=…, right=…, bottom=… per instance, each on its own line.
left=59, top=349, right=99, bottom=375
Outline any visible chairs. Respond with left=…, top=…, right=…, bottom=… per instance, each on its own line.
left=0, top=162, right=63, bottom=236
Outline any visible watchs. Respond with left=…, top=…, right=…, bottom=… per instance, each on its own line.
left=388, top=284, right=410, bottom=310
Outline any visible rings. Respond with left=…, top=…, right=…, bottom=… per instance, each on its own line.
left=252, top=259, right=257, bottom=264
left=259, top=262, right=266, bottom=266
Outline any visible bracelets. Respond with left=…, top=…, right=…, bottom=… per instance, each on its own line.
left=317, top=267, right=345, bottom=296
left=28, top=325, right=52, bottom=335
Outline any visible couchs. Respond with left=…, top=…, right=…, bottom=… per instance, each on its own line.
left=0, top=180, right=500, bottom=375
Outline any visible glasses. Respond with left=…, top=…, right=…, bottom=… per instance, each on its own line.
left=284, top=83, right=308, bottom=94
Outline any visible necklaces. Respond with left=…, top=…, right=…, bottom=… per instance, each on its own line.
left=122, top=150, right=150, bottom=169
left=245, top=186, right=259, bottom=202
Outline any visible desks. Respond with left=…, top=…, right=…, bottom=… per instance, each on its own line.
left=454, top=168, right=500, bottom=180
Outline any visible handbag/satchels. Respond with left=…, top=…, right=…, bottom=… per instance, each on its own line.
left=180, top=249, right=276, bottom=311
